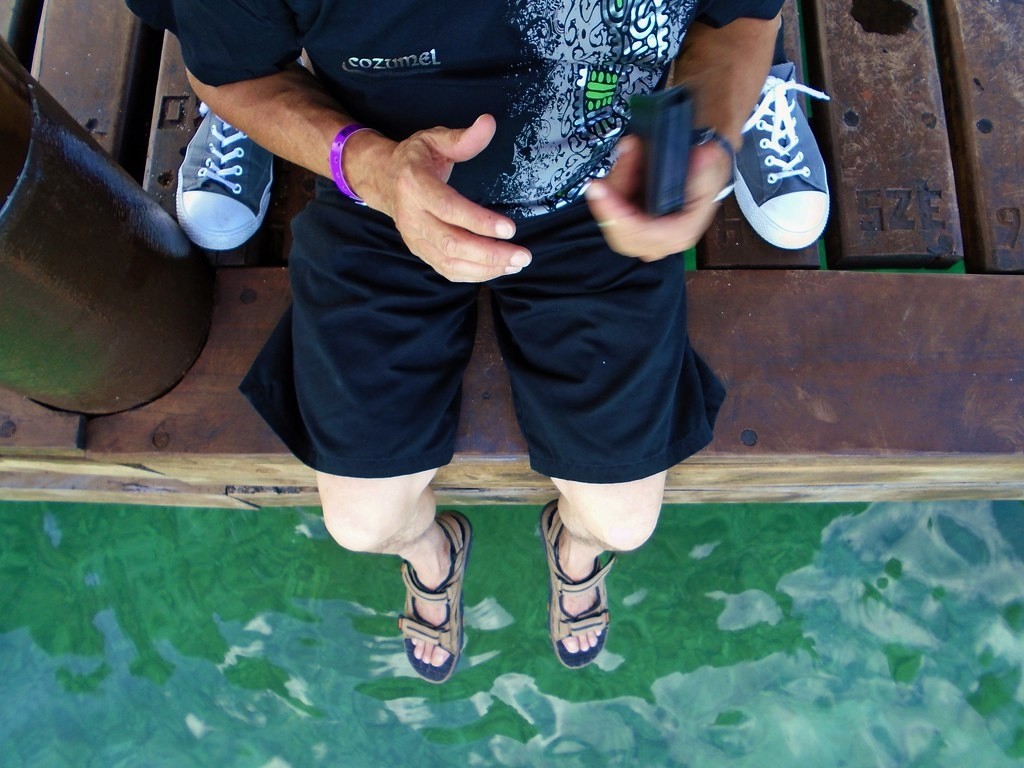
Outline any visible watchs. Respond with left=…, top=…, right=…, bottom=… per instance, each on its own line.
left=696, top=127, right=740, bottom=204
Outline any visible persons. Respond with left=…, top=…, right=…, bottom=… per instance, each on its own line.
left=125, top=0, right=830, bottom=686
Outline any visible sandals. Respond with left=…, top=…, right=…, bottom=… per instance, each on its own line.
left=398, top=507, right=473, bottom=684
left=542, top=497, right=616, bottom=669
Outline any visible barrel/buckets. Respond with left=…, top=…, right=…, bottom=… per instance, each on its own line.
left=0, top=55, right=214, bottom=417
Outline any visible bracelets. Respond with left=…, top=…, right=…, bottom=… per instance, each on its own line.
left=328, top=122, right=387, bottom=207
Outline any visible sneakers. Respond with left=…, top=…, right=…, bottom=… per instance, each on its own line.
left=732, top=61, right=830, bottom=249
left=176, top=102, right=274, bottom=251
